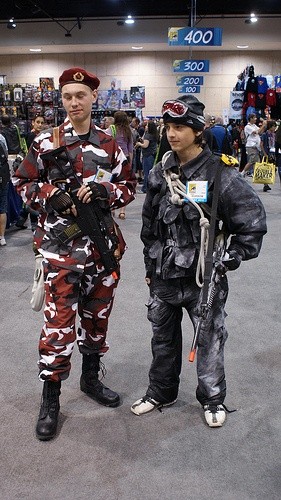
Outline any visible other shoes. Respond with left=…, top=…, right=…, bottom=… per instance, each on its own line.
left=119, top=213, right=125, bottom=218
left=241, top=173, right=246, bottom=180
left=137, top=190, right=144, bottom=194
left=264, top=186, right=271, bottom=192
left=111, top=211, right=115, bottom=217
left=32, top=226, right=36, bottom=232
left=137, top=177, right=142, bottom=181
left=15, top=224, right=27, bottom=229
left=0, top=239, right=6, bottom=246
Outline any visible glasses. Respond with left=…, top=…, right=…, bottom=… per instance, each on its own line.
left=162, top=100, right=191, bottom=117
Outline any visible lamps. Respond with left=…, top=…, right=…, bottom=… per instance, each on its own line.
left=245, top=15, right=253, bottom=24
left=10, top=17, right=15, bottom=22
left=65, top=32, right=72, bottom=37
left=117, top=19, right=125, bottom=26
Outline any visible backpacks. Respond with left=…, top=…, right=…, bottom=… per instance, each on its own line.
left=241, top=125, right=249, bottom=144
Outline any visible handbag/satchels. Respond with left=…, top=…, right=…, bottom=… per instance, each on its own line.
left=14, top=124, right=28, bottom=154
left=30, top=258, right=47, bottom=312
left=222, top=129, right=232, bottom=155
left=253, top=156, right=276, bottom=184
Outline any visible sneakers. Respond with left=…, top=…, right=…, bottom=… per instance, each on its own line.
left=203, top=403, right=237, bottom=427
left=130, top=393, right=177, bottom=415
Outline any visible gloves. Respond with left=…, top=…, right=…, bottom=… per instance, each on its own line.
left=223, top=258, right=239, bottom=270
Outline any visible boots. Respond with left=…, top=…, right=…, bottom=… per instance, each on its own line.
left=79, top=353, right=119, bottom=407
left=36, top=379, right=62, bottom=439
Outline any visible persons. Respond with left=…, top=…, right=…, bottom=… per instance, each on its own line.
left=0, top=116, right=46, bottom=246
left=101, top=110, right=172, bottom=220
left=204, top=105, right=281, bottom=192
left=131, top=96, right=268, bottom=428
left=10, top=68, right=138, bottom=441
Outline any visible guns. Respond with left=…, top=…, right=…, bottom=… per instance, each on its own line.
left=188, top=233, right=225, bottom=363
left=39, top=146, right=119, bottom=280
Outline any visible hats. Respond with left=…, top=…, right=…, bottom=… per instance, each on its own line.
left=163, top=95, right=206, bottom=129
left=59, top=67, right=100, bottom=91
